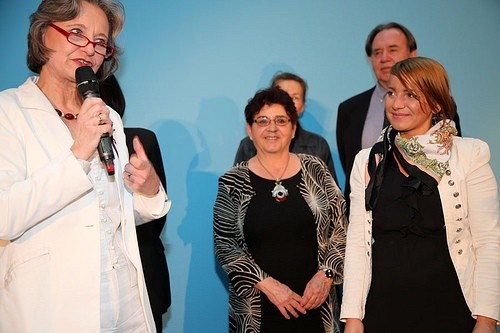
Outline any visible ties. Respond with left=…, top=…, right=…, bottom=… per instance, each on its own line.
left=382, top=108, right=391, bottom=129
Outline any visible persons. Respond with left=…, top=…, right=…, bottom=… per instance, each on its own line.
left=213, top=85, right=348, bottom=333
left=339, top=56, right=500, bottom=333
left=0, top=0, right=171, bottom=333
left=98, top=72, right=171, bottom=333
left=336, top=22, right=462, bottom=220
left=233, top=70, right=342, bottom=190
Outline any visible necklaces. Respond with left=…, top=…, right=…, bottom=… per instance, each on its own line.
left=56, top=108, right=80, bottom=120
left=257, top=153, right=290, bottom=202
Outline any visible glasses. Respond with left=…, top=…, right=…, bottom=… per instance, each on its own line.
left=252, top=115, right=290, bottom=127
left=47, top=22, right=115, bottom=57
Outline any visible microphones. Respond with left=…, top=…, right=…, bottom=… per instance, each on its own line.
left=76, top=66, right=115, bottom=176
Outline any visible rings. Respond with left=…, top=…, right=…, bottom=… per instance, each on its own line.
left=98, top=115, right=102, bottom=123
left=128, top=174, right=132, bottom=181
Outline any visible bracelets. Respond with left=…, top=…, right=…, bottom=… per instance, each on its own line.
left=323, top=269, right=334, bottom=279
